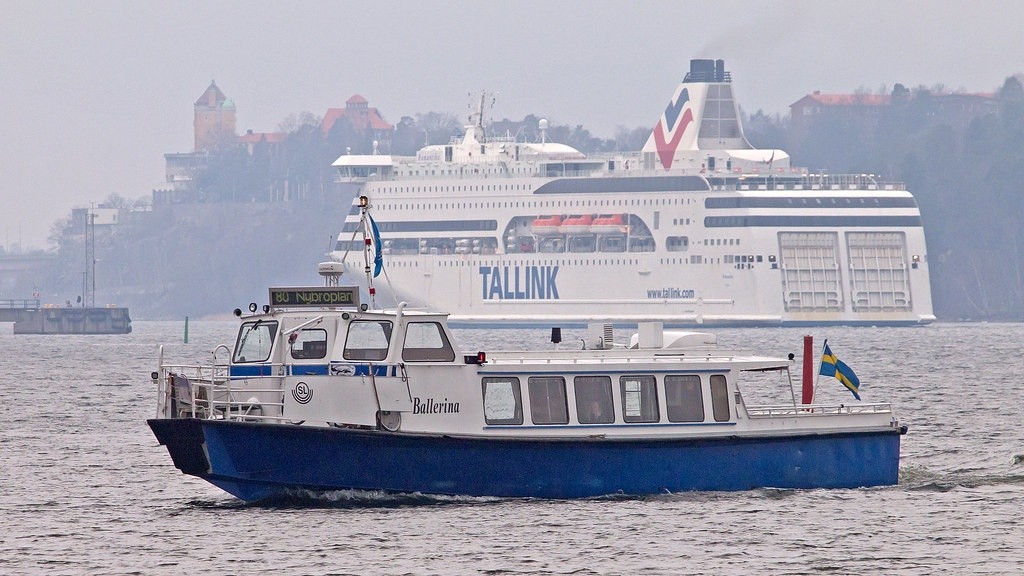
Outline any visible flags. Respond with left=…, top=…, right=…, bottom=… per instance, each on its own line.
left=364, top=209, right=382, bottom=278
left=821, top=339, right=862, bottom=401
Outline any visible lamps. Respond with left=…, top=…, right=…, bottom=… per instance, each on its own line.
left=361, top=304, right=368, bottom=311
left=263, top=305, right=269, bottom=314
left=249, top=303, right=257, bottom=314
left=342, top=313, right=349, bottom=320
left=234, top=308, right=242, bottom=317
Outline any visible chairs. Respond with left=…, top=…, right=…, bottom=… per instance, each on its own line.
left=172, top=373, right=219, bottom=421
left=198, top=386, right=265, bottom=424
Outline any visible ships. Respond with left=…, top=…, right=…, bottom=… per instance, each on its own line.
left=309, top=56, right=939, bottom=328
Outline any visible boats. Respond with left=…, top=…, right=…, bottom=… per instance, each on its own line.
left=562, top=215, right=592, bottom=237
left=530, top=216, right=561, bottom=235
left=591, top=214, right=628, bottom=235
left=146, top=196, right=908, bottom=511
